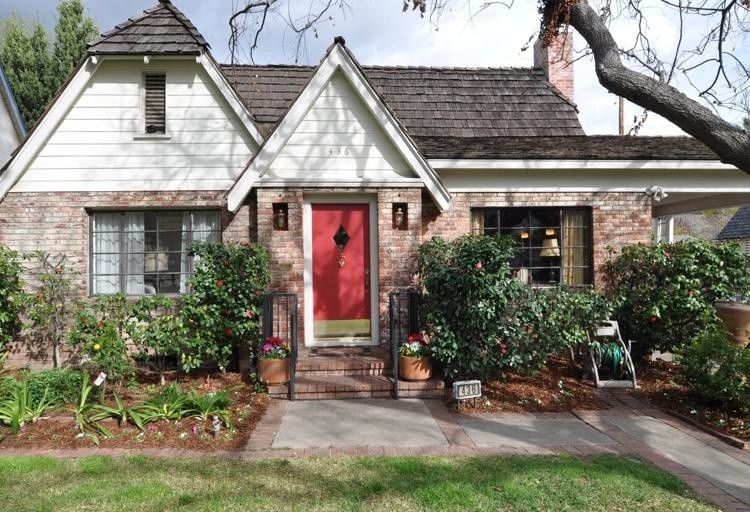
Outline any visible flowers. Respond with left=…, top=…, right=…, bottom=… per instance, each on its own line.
left=397, top=334, right=430, bottom=357
left=256, top=337, right=289, bottom=359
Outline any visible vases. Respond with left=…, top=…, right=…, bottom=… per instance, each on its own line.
left=398, top=355, right=432, bottom=382
left=256, top=359, right=290, bottom=385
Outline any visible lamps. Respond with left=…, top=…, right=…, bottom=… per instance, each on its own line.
left=394, top=208, right=404, bottom=230
left=145, top=253, right=168, bottom=289
left=539, top=239, right=560, bottom=284
left=276, top=210, right=287, bottom=230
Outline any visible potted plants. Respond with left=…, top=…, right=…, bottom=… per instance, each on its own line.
left=715, top=237, right=750, bottom=348
